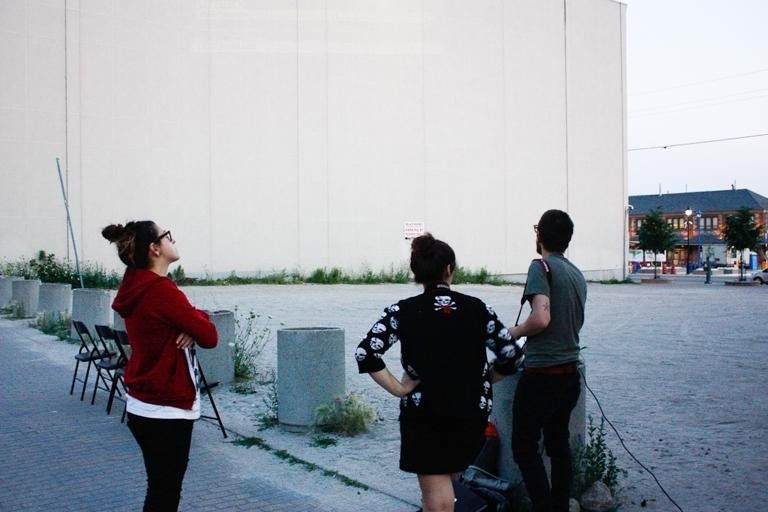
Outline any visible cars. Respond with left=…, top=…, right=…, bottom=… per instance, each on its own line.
left=753, top=268, right=768, bottom=284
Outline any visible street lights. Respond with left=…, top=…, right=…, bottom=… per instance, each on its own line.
left=696, top=209, right=702, bottom=268
left=685, top=207, right=692, bottom=275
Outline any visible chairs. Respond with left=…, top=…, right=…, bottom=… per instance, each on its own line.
left=69, top=321, right=229, bottom=440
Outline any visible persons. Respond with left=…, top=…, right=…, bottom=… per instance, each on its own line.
left=354, top=231, right=523, bottom=511
left=100, top=219, right=219, bottom=512
left=506, top=209, right=589, bottom=512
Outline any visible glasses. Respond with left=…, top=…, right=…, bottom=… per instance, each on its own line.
left=152, top=230, right=174, bottom=244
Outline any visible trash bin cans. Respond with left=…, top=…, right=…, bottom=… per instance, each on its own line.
left=631, top=261, right=640, bottom=273
left=687, top=263, right=694, bottom=274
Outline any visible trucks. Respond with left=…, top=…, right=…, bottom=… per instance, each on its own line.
left=702, top=244, right=758, bottom=269
